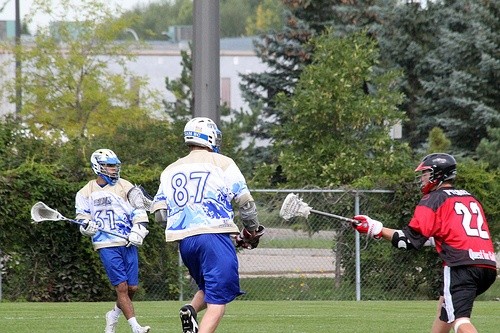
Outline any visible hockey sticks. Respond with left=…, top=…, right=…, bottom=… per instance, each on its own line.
left=279, top=193, right=361, bottom=224
left=126, top=185, right=154, bottom=211
left=30, top=201, right=128, bottom=241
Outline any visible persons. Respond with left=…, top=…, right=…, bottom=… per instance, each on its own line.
left=353, top=153, right=498, bottom=333
left=75, top=149, right=152, bottom=333
left=149, top=117, right=266, bottom=333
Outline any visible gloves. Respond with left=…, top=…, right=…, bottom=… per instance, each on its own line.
left=352, top=214, right=382, bottom=242
left=235, top=225, right=265, bottom=250
left=128, top=225, right=150, bottom=248
left=80, top=219, right=98, bottom=238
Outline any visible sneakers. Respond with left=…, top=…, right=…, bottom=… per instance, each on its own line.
left=133, top=326, right=150, bottom=333
left=179, top=305, right=200, bottom=333
left=105, top=311, right=118, bottom=333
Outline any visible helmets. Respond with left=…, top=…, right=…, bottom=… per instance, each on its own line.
left=90, top=149, right=121, bottom=186
left=183, top=116, right=222, bottom=153
left=415, top=153, right=457, bottom=194
